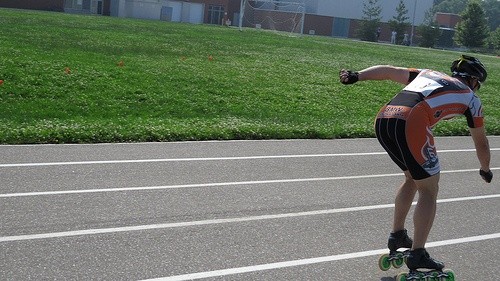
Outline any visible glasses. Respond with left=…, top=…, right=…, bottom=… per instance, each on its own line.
left=477, top=81, right=482, bottom=90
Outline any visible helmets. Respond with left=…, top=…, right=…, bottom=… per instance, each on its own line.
left=451, top=55, right=487, bottom=82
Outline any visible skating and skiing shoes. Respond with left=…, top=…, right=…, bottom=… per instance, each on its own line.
left=378, top=229, right=413, bottom=271
left=396, top=249, right=456, bottom=281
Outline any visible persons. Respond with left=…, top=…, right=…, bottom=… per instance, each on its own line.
left=403, top=31, right=410, bottom=46
left=339, top=54, right=493, bottom=271
left=391, top=30, right=397, bottom=44
left=377, top=23, right=381, bottom=40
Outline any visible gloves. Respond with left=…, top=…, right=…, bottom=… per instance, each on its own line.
left=338, top=68, right=358, bottom=84
left=479, top=169, right=493, bottom=183
